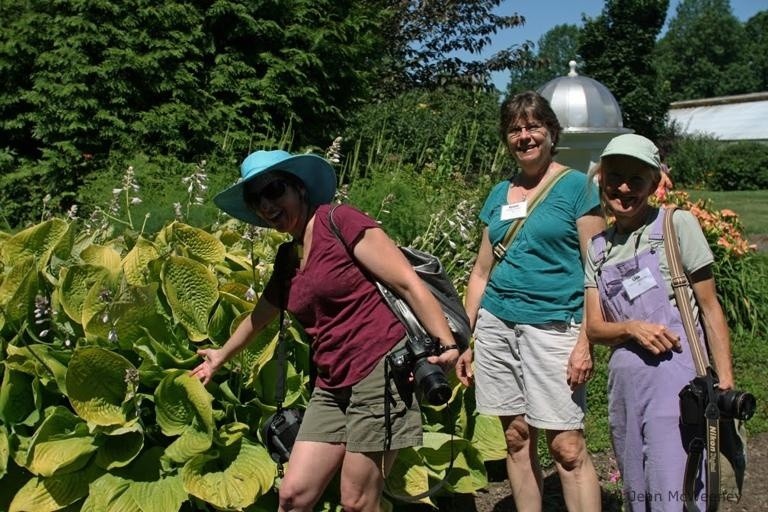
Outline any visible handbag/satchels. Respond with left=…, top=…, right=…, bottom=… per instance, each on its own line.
left=376, top=239, right=472, bottom=359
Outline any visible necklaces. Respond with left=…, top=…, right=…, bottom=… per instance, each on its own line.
left=294, top=201, right=310, bottom=258
left=519, top=175, right=548, bottom=202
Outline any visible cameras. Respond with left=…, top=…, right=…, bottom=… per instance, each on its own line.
left=388, top=335, right=452, bottom=405
left=261, top=409, right=302, bottom=466
left=679, top=379, right=756, bottom=427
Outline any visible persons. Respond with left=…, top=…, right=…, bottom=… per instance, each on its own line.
left=186, top=149, right=461, bottom=512
left=584, top=132, right=737, bottom=512
left=455, top=91, right=606, bottom=512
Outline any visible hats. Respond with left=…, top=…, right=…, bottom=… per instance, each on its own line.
left=212, top=148, right=341, bottom=228
left=598, top=133, right=663, bottom=169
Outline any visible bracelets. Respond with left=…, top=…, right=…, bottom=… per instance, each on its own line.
left=438, top=342, right=458, bottom=354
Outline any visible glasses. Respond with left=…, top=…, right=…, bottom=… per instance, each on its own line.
left=505, top=122, right=548, bottom=137
left=246, top=177, right=290, bottom=210
